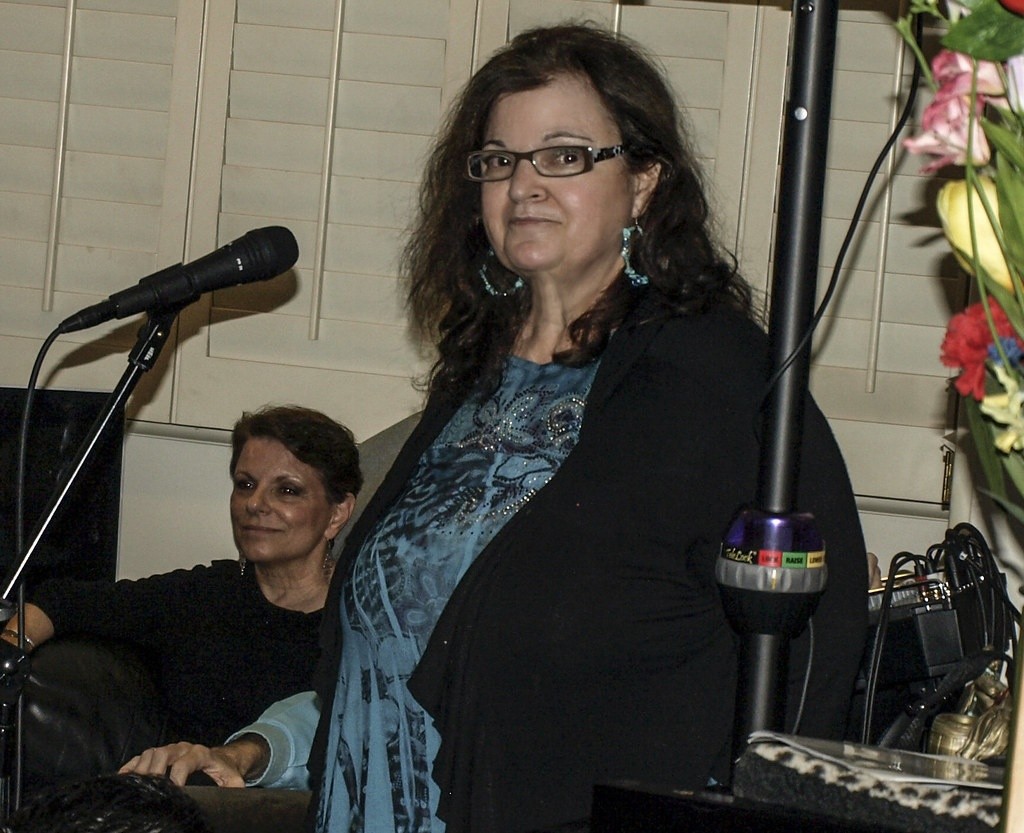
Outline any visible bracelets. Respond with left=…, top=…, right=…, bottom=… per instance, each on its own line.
left=2, top=628, right=34, bottom=649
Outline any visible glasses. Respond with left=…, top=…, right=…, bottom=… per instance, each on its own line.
left=462, top=144, right=625, bottom=183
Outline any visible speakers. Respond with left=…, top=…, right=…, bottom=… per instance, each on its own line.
left=0, top=386, right=124, bottom=606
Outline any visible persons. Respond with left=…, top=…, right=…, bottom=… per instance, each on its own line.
left=0, top=405, right=364, bottom=784
left=307, top=27, right=869, bottom=833
left=117, top=553, right=322, bottom=791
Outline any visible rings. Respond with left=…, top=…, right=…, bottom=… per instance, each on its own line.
left=151, top=747, right=156, bottom=751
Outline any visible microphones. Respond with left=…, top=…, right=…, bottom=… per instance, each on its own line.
left=58, top=226, right=300, bottom=334
